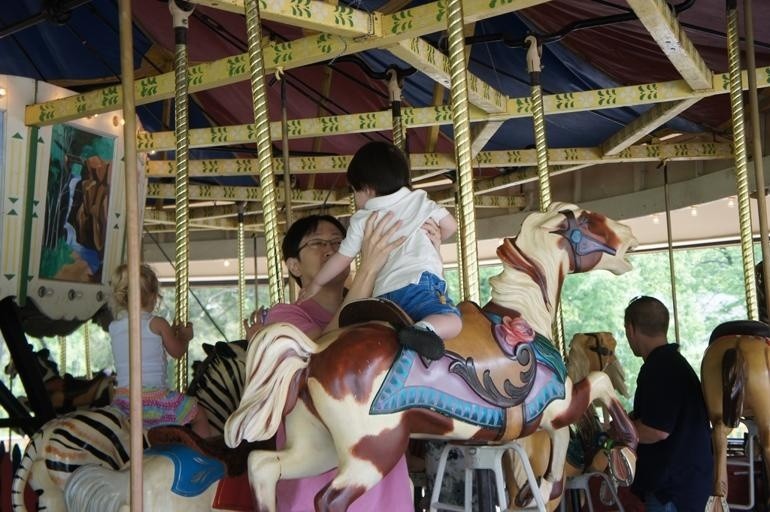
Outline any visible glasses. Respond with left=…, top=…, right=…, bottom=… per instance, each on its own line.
left=297, top=239, right=341, bottom=253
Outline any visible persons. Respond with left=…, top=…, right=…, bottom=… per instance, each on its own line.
left=264, top=210, right=441, bottom=512
left=296, top=142, right=463, bottom=359
left=108, top=264, right=209, bottom=439
left=610, top=297, right=713, bottom=512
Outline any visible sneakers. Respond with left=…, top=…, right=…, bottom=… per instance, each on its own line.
left=398, top=326, right=444, bottom=360
